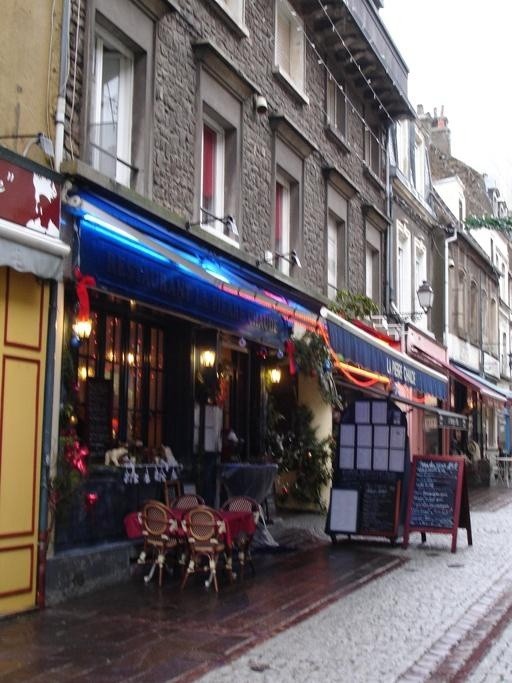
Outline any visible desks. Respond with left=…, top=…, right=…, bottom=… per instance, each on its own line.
left=128, top=508, right=255, bottom=584
left=57, top=465, right=182, bottom=548
left=222, top=462, right=278, bottom=506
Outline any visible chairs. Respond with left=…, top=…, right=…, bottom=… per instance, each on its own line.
left=139, top=494, right=260, bottom=592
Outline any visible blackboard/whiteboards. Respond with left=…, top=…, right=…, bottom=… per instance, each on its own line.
left=324, top=478, right=400, bottom=537
left=404, top=454, right=463, bottom=532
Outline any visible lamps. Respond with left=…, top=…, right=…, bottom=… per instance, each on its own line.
left=186, top=213, right=300, bottom=270
left=0, top=132, right=55, bottom=158
left=401, top=280, right=434, bottom=315
